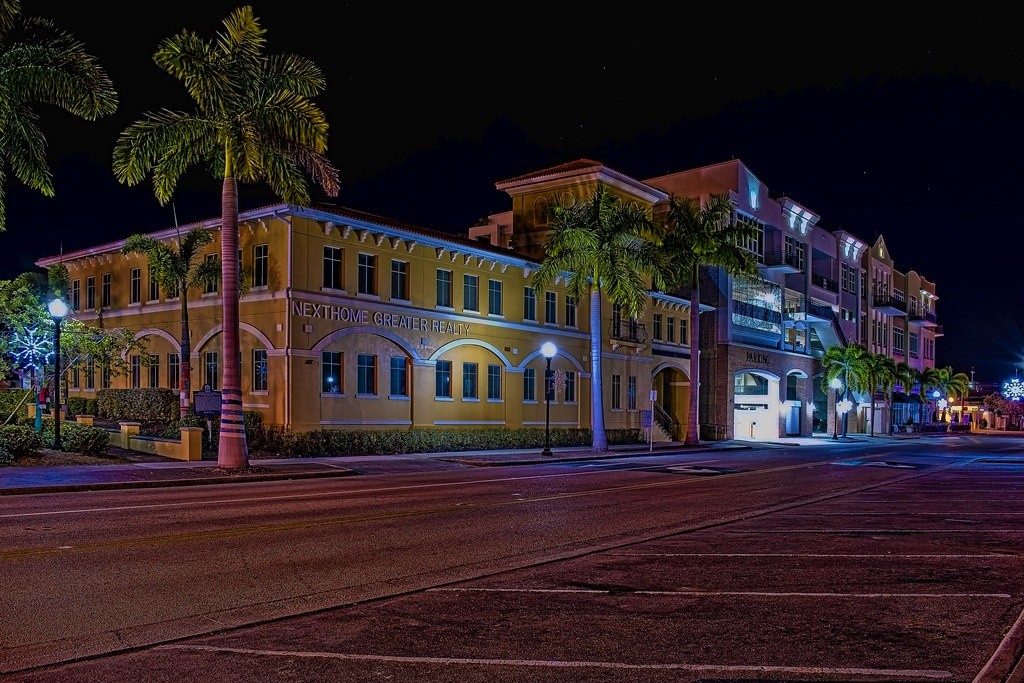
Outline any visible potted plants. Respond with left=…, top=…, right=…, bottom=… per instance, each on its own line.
left=903, top=417, right=916, bottom=433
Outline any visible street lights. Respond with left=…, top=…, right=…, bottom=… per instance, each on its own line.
left=948, top=397, right=954, bottom=416
left=541, top=341, right=557, bottom=456
left=49, top=298, right=68, bottom=452
left=831, top=380, right=841, bottom=440
left=933, top=391, right=941, bottom=425
